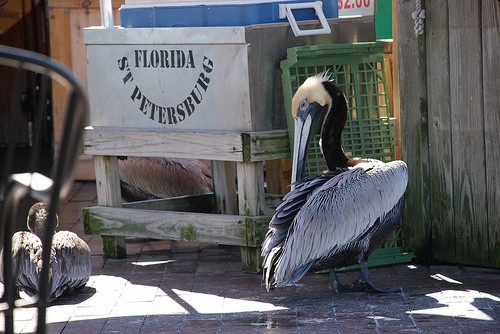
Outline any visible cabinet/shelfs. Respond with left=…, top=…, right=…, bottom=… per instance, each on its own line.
left=83, top=15, right=375, bottom=273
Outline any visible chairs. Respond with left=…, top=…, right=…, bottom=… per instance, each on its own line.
left=0, top=44, right=89, bottom=334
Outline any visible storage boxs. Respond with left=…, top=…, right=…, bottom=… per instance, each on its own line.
left=119, top=0, right=339, bottom=37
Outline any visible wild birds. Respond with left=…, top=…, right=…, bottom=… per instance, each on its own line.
left=117, top=156, right=214, bottom=202
left=0, top=201, right=92, bottom=303
left=260, top=68, right=410, bottom=293
